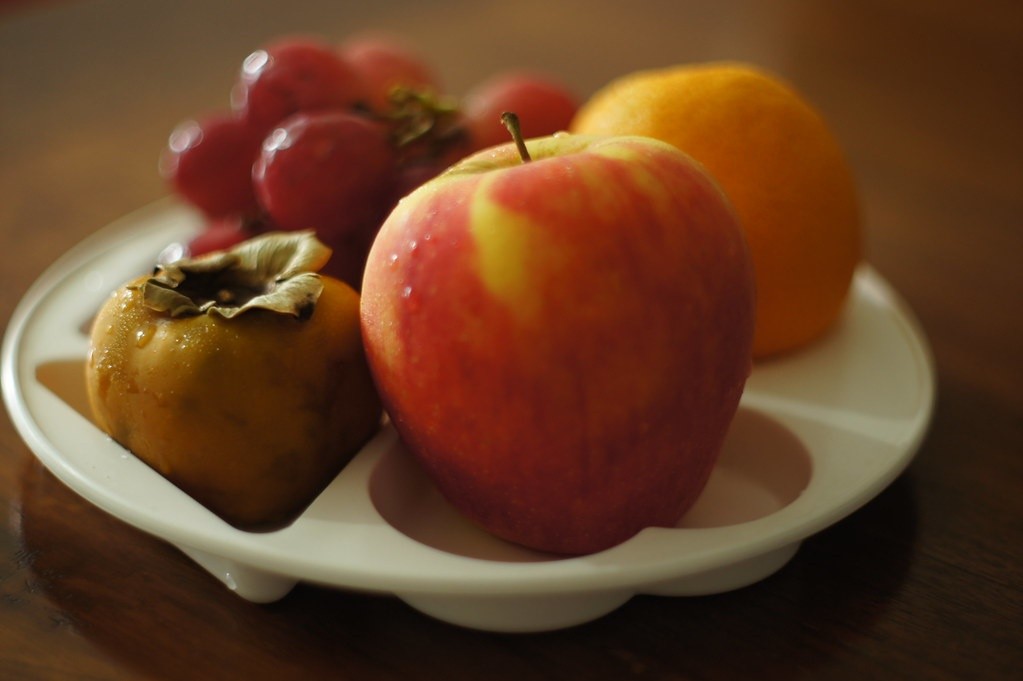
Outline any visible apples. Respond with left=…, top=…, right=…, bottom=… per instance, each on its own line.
left=358, top=110, right=756, bottom=563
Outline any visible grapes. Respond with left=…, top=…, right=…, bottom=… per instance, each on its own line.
left=165, top=40, right=579, bottom=256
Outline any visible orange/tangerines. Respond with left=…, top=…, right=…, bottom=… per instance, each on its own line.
left=567, top=62, right=867, bottom=361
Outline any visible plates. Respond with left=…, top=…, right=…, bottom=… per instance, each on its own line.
left=0, top=189, right=937, bottom=635
